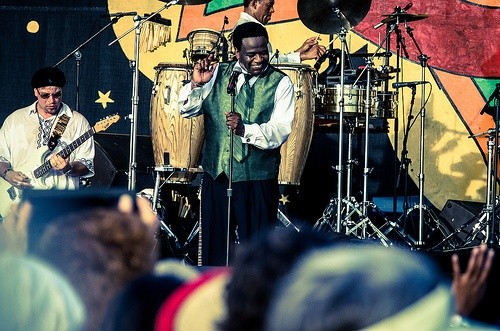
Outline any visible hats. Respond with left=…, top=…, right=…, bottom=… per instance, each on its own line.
left=32, top=66, right=66, bottom=88
left=262, top=239, right=444, bottom=330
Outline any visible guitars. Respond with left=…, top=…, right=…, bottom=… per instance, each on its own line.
left=0, top=113, right=120, bottom=219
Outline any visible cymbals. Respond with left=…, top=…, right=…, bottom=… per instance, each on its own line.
left=296, top=0, right=372, bottom=35
left=379, top=10, right=429, bottom=23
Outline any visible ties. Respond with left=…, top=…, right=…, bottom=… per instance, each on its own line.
left=232, top=74, right=253, bottom=163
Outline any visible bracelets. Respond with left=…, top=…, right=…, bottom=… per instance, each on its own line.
left=191, top=79, right=205, bottom=87
left=2, top=168, right=13, bottom=180
left=63, top=163, right=73, bottom=178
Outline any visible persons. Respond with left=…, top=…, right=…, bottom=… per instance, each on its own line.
left=0, top=66, right=95, bottom=224
left=177, top=22, right=295, bottom=267
left=227, top=0, right=326, bottom=65
left=0, top=194, right=495, bottom=331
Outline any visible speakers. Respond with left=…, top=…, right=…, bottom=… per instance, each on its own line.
left=78, top=133, right=156, bottom=191
left=423, top=199, right=500, bottom=279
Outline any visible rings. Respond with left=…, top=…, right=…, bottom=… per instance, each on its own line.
left=55, top=164, right=58, bottom=167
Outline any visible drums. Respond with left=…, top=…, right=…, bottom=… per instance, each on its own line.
left=374, top=89, right=399, bottom=122
left=186, top=28, right=227, bottom=63
left=148, top=62, right=204, bottom=186
left=258, top=59, right=317, bottom=188
left=313, top=83, right=380, bottom=119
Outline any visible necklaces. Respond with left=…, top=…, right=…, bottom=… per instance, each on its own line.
left=37, top=109, right=60, bottom=138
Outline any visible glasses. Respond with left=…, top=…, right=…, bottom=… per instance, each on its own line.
left=36, top=87, right=62, bottom=99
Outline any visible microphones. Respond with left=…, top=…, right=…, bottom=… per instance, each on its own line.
left=224, top=16, right=229, bottom=24
left=393, top=81, right=429, bottom=88
left=226, top=67, right=242, bottom=94
left=100, top=12, right=137, bottom=18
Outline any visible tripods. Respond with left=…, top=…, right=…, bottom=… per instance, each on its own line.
left=314, top=11, right=420, bottom=252
